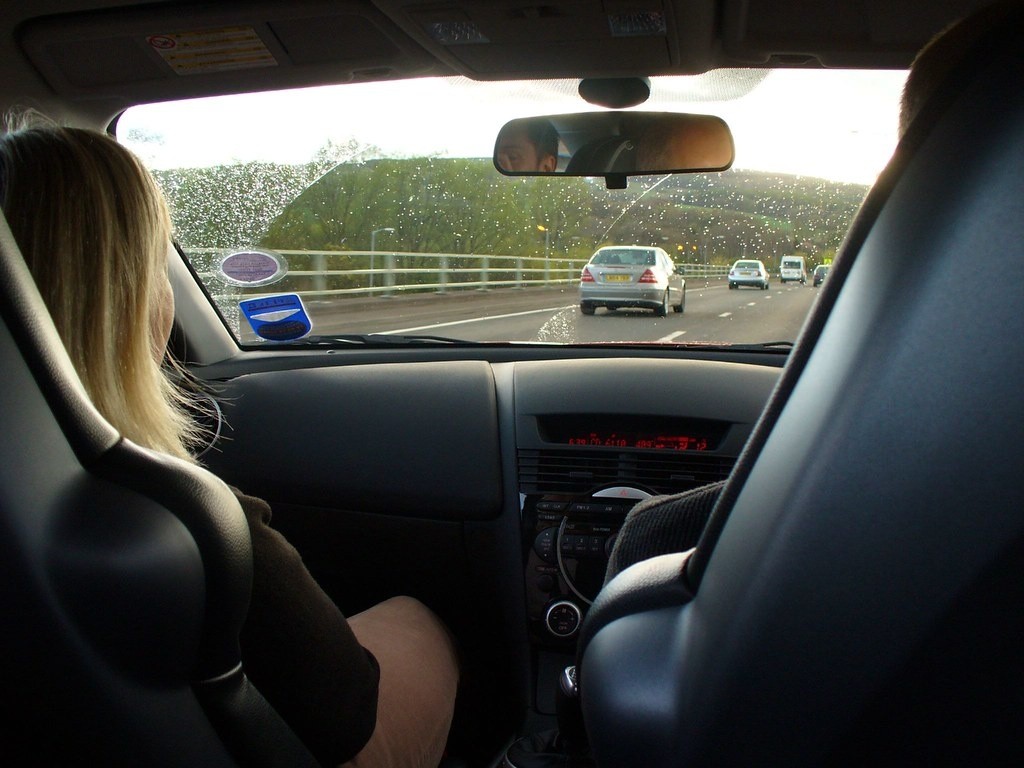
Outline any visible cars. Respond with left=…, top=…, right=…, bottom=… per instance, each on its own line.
left=580, top=244, right=687, bottom=316
left=728, top=259, right=770, bottom=290
left=813, top=265, right=831, bottom=287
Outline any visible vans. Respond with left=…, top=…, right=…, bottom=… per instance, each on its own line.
left=779, top=255, right=807, bottom=285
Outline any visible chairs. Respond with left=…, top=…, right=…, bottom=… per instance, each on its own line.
left=0, top=207, right=280, bottom=768
left=547, top=2, right=1024, bottom=768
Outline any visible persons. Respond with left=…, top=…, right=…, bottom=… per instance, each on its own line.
left=494, top=118, right=563, bottom=176
left=634, top=113, right=732, bottom=171
left=603, top=0, right=1023, bottom=583
left=0, top=112, right=473, bottom=768
